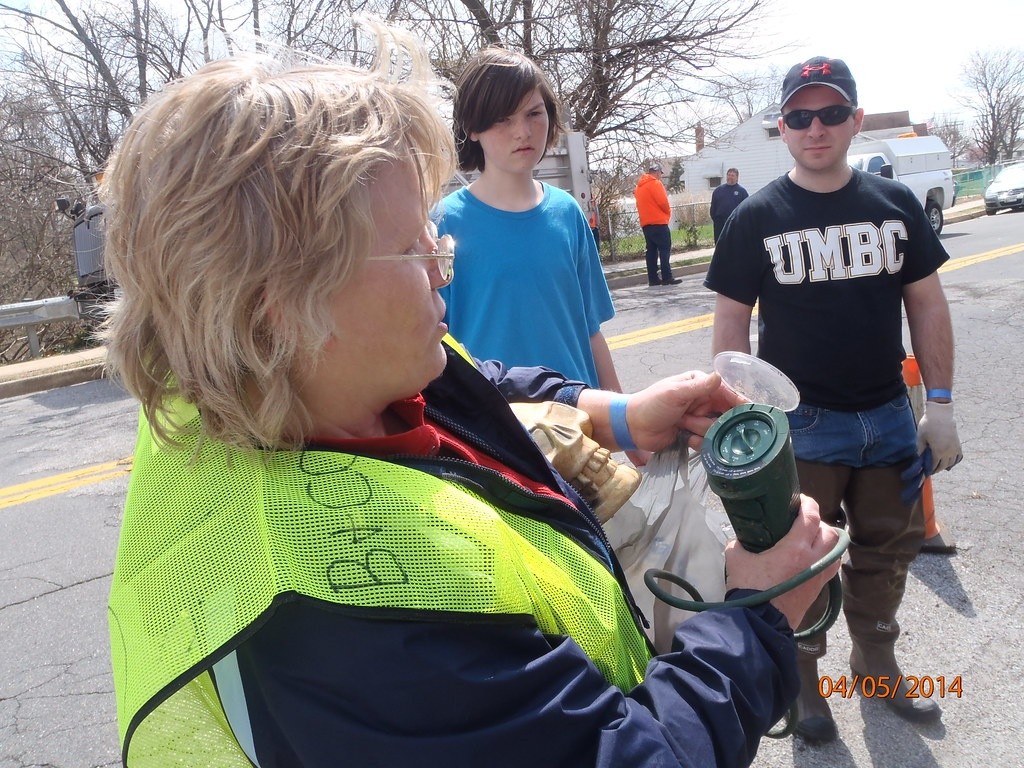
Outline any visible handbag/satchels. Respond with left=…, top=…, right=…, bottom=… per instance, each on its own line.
left=603, top=430, right=735, bottom=655
left=508, top=400, right=643, bottom=525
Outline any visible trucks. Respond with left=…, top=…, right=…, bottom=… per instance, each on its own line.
left=827, top=136, right=955, bottom=242
left=54, top=131, right=601, bottom=324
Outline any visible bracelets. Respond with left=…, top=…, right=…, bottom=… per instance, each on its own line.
left=926, top=387, right=952, bottom=401
left=607, top=395, right=637, bottom=449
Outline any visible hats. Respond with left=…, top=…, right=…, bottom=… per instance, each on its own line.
left=649, top=162, right=665, bottom=174
left=780, top=56, right=856, bottom=109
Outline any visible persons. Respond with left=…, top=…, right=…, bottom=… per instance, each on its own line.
left=708, top=57, right=964, bottom=746
left=429, top=44, right=624, bottom=394
left=635, top=164, right=683, bottom=285
left=95, top=51, right=843, bottom=768
left=709, top=167, right=749, bottom=248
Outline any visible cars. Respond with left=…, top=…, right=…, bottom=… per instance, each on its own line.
left=983, top=163, right=1024, bottom=216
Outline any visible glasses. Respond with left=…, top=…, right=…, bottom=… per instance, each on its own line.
left=783, top=104, right=855, bottom=129
left=327, top=234, right=454, bottom=281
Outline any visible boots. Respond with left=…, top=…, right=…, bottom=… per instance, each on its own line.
left=840, top=462, right=939, bottom=720
left=784, top=459, right=846, bottom=740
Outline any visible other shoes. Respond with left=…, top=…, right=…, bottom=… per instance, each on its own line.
left=649, top=277, right=662, bottom=286
left=662, top=276, right=682, bottom=285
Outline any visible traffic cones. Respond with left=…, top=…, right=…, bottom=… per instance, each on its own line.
left=898, top=354, right=960, bottom=554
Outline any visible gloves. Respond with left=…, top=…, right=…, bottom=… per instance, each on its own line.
left=900, top=449, right=932, bottom=507
left=914, top=401, right=962, bottom=475
